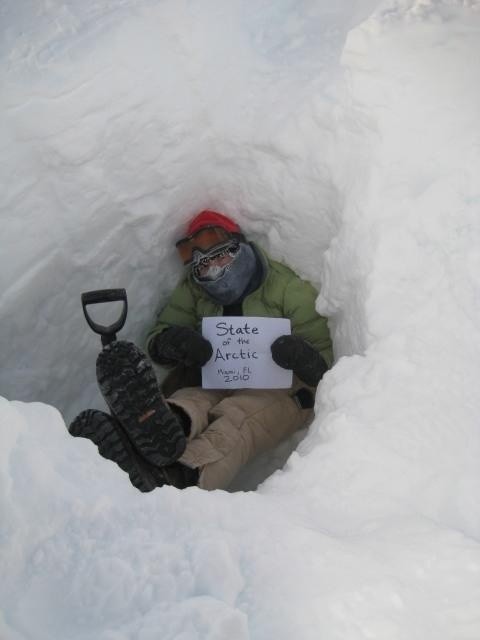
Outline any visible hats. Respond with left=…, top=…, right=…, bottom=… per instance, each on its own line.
left=186, top=210, right=239, bottom=232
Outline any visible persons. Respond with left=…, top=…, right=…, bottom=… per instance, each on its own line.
left=66, top=207, right=335, bottom=492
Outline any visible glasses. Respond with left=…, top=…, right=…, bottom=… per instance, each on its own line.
left=176, top=226, right=238, bottom=268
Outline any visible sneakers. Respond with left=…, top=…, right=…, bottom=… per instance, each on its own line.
left=67, top=409, right=166, bottom=493
left=94, top=339, right=187, bottom=468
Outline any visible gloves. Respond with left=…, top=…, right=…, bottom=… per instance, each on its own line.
left=157, top=326, right=213, bottom=367
left=271, top=335, right=327, bottom=386
